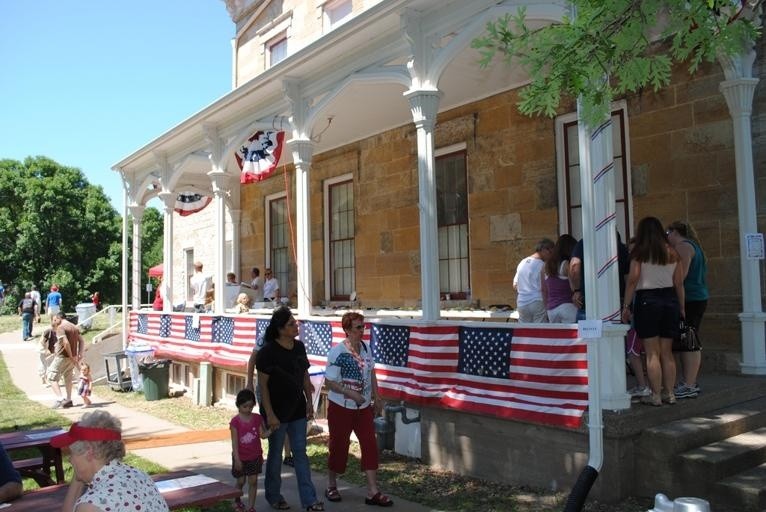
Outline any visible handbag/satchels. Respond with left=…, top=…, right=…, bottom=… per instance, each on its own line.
left=672, top=318, right=703, bottom=352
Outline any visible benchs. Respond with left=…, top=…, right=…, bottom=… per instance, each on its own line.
left=11, top=455, right=52, bottom=470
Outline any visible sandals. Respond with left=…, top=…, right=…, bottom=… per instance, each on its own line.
left=272, top=500, right=290, bottom=510
left=248, top=505, right=256, bottom=512
left=233, top=501, right=247, bottom=512
left=307, top=502, right=325, bottom=512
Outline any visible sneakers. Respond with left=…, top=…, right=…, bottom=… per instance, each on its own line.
left=52, top=398, right=65, bottom=409
left=63, top=400, right=72, bottom=407
left=626, top=385, right=652, bottom=397
left=662, top=393, right=676, bottom=404
left=674, top=387, right=698, bottom=399
left=673, top=382, right=700, bottom=392
left=641, top=395, right=662, bottom=406
left=283, top=457, right=294, bottom=466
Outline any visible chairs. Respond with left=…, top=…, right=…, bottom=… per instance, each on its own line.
left=481, top=305, right=514, bottom=322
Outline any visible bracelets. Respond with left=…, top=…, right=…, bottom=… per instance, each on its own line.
left=622, top=303, right=631, bottom=309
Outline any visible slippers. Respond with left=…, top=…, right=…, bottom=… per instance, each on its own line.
left=365, top=492, right=393, bottom=506
left=325, top=487, right=342, bottom=502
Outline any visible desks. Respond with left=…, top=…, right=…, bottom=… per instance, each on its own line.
left=0, top=468, right=243, bottom=512
left=0, top=425, right=70, bottom=483
left=227, top=306, right=520, bottom=322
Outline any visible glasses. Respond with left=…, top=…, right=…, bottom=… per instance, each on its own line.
left=264, top=273, right=271, bottom=276
left=350, top=325, right=365, bottom=329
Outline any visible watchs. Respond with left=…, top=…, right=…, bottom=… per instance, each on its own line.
left=571, top=288, right=580, bottom=293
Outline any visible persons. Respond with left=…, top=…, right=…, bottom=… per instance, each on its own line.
left=0, top=283, right=10, bottom=316
left=229, top=389, right=273, bottom=512
left=46, top=285, right=63, bottom=322
left=226, top=272, right=237, bottom=285
left=50, top=409, right=169, bottom=512
left=234, top=291, right=250, bottom=314
left=30, top=284, right=42, bottom=323
left=263, top=268, right=280, bottom=302
left=621, top=215, right=686, bottom=406
left=190, top=261, right=214, bottom=313
left=72, top=359, right=93, bottom=409
left=256, top=307, right=325, bottom=512
left=18, top=292, right=39, bottom=341
left=568, top=229, right=625, bottom=324
left=91, top=291, right=99, bottom=313
left=512, top=238, right=554, bottom=322
left=46, top=311, right=84, bottom=409
left=324, top=313, right=394, bottom=506
left=36, top=313, right=57, bottom=388
left=541, top=234, right=579, bottom=323
left=246, top=318, right=314, bottom=467
left=240, top=268, right=265, bottom=302
left=151, top=276, right=164, bottom=311
left=0, top=440, right=23, bottom=502
left=667, top=219, right=708, bottom=400
left=623, top=312, right=654, bottom=397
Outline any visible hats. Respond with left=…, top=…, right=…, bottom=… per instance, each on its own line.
left=50, top=423, right=121, bottom=448
